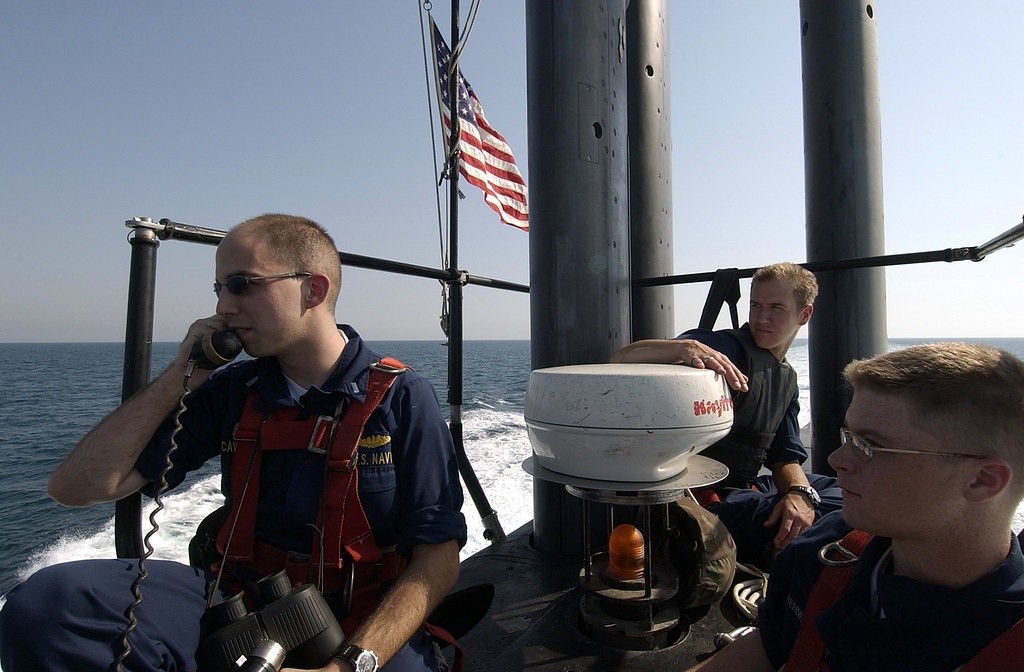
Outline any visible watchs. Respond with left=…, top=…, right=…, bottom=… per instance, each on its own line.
left=335, top=643, right=380, bottom=671
left=787, top=486, right=822, bottom=509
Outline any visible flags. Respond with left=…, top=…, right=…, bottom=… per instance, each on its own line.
left=428, top=10, right=530, bottom=230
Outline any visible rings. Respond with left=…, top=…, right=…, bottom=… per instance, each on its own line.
left=691, top=356, right=698, bottom=367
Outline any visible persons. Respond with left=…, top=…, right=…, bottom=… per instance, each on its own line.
left=609, top=265, right=844, bottom=572
left=0, top=212, right=467, bottom=672
left=757, top=344, right=1024, bottom=672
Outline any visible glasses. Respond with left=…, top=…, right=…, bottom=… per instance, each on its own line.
left=213, top=272, right=315, bottom=297
left=840, top=422, right=990, bottom=464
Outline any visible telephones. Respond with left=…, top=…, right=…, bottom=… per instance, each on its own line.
left=188, top=327, right=243, bottom=371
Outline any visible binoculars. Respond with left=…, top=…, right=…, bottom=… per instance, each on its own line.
left=194, top=568, right=350, bottom=672
